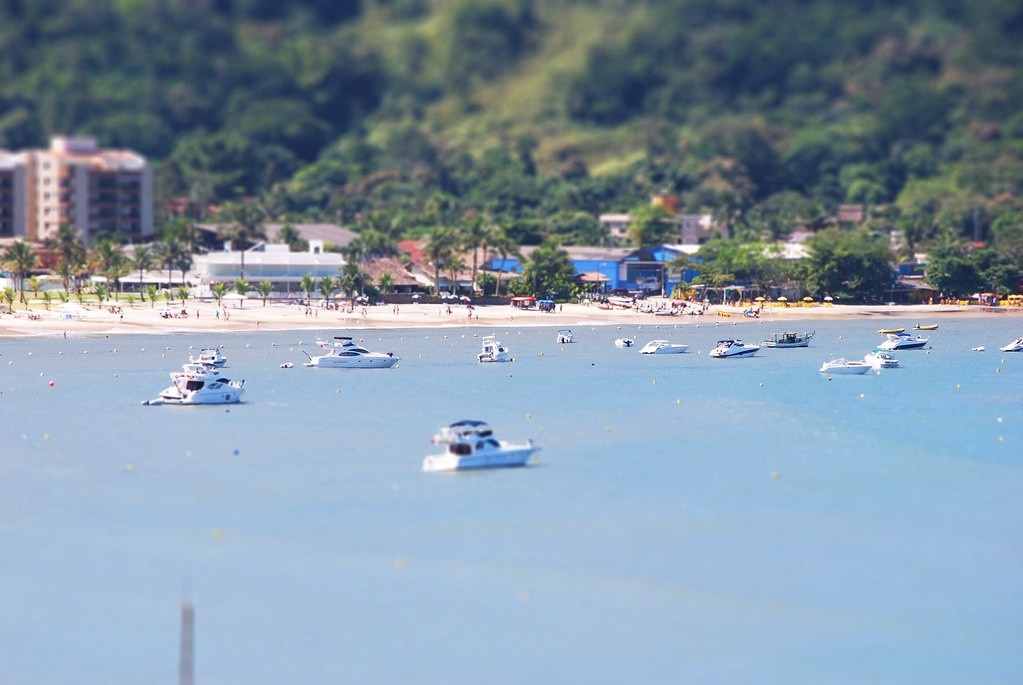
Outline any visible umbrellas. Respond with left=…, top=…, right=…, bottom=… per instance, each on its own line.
left=355, top=297, right=368, bottom=305
left=186, top=287, right=248, bottom=308
left=410, top=294, right=471, bottom=305
left=754, top=295, right=833, bottom=303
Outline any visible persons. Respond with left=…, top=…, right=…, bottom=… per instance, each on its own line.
left=160, top=303, right=260, bottom=330
left=750, top=303, right=765, bottom=318
left=305, top=302, right=399, bottom=319
left=510, top=290, right=636, bottom=312
left=28, top=298, right=123, bottom=339
left=447, top=305, right=473, bottom=320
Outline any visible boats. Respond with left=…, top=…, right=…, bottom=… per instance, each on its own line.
left=639, top=340, right=690, bottom=354
left=141, top=348, right=246, bottom=405
left=1000, top=337, right=1023, bottom=352
left=478, top=336, right=510, bottom=363
left=761, top=331, right=816, bottom=348
left=709, top=339, right=760, bottom=358
left=557, top=329, right=574, bottom=343
left=302, top=340, right=401, bottom=368
left=421, top=420, right=542, bottom=472
left=615, top=339, right=634, bottom=347
left=819, top=323, right=939, bottom=374
